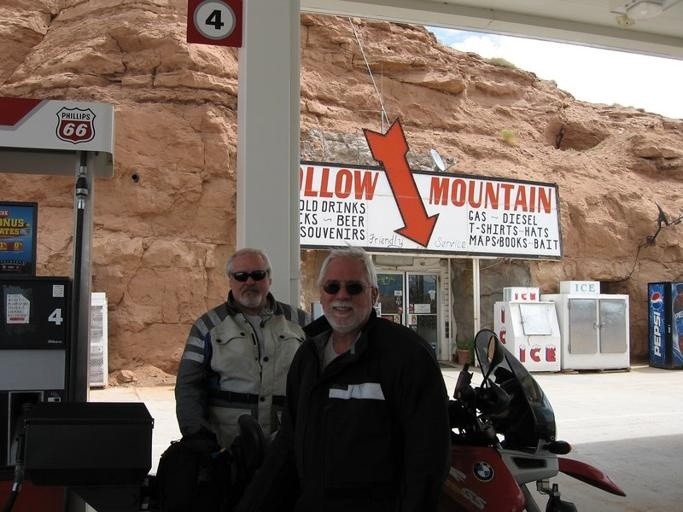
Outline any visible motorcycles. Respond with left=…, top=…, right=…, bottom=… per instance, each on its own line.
left=440, top=328, right=629, bottom=512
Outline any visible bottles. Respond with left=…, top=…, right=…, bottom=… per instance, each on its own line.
left=673, top=285, right=683, bottom=352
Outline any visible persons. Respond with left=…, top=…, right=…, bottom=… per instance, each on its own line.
left=174, top=248, right=311, bottom=495
left=243, top=248, right=449, bottom=512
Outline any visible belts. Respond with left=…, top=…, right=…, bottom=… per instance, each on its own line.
left=215, top=390, right=287, bottom=405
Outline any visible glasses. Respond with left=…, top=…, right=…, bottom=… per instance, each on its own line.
left=230, top=269, right=268, bottom=282
left=323, top=280, right=373, bottom=296
